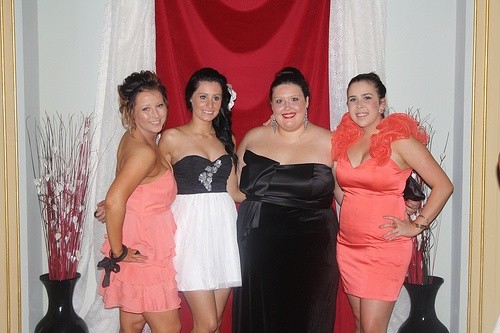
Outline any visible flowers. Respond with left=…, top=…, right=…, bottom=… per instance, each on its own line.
left=227, top=83, right=237, bottom=111
left=387, top=107, right=450, bottom=284
left=26, top=110, right=114, bottom=281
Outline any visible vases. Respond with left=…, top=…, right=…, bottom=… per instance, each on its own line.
left=34, top=272, right=89, bottom=333
left=397, top=275, right=450, bottom=333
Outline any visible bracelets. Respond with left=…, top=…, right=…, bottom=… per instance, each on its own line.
left=411, top=222, right=428, bottom=230
left=418, top=215, right=429, bottom=225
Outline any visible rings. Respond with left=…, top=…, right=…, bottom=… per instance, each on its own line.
left=135, top=250, right=140, bottom=255
left=94, top=211, right=98, bottom=217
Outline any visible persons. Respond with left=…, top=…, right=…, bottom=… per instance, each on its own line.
left=97, top=70, right=181, bottom=333
left=94, top=67, right=246, bottom=333
left=331, top=72, right=454, bottom=333
left=236, top=68, right=420, bottom=333
